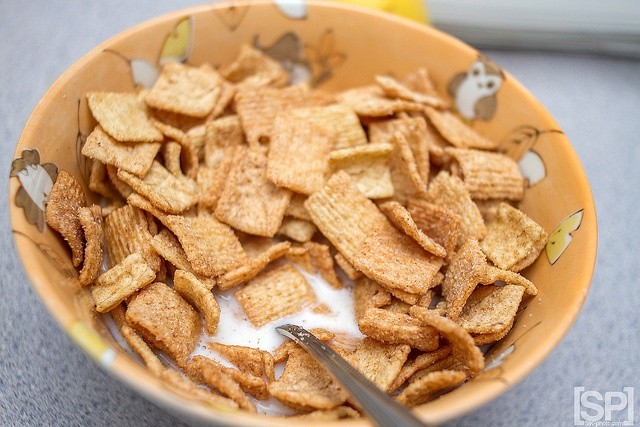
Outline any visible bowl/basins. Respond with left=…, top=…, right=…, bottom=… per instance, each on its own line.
left=9, top=1, right=597, bottom=426
